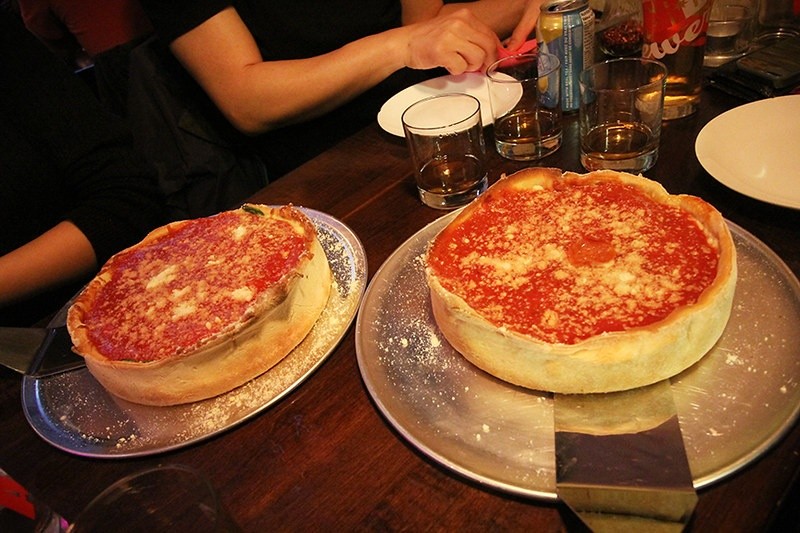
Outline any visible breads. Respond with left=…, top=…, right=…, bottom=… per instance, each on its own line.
left=65, top=203, right=333, bottom=405
left=422, top=165, right=738, bottom=394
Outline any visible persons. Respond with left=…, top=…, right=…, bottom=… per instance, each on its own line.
left=15, top=0, right=546, bottom=222
left=0, top=0, right=136, bottom=326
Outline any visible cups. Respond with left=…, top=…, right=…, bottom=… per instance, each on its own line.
left=486, top=52, right=564, bottom=161
left=402, top=93, right=490, bottom=210
left=595, top=1, right=645, bottom=55
left=577, top=57, right=668, bottom=174
left=757, top=0, right=800, bottom=44
left=636, top=0, right=712, bottom=119
left=65, top=464, right=244, bottom=533
left=702, top=0, right=762, bottom=67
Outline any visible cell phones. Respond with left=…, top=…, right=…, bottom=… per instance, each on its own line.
left=703, top=34, right=800, bottom=102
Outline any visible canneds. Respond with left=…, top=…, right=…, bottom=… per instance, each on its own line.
left=536, top=0, right=597, bottom=112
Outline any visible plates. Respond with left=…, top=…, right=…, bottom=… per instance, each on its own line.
left=353, top=204, right=799, bottom=501
left=376, top=71, right=524, bottom=139
left=694, top=94, right=800, bottom=208
left=20, top=206, right=369, bottom=458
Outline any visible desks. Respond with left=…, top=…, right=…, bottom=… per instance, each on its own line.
left=0, top=78, right=800, bottom=533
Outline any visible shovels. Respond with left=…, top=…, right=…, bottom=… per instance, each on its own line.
left=553, top=378, right=700, bottom=533
left=0, top=325, right=87, bottom=379
